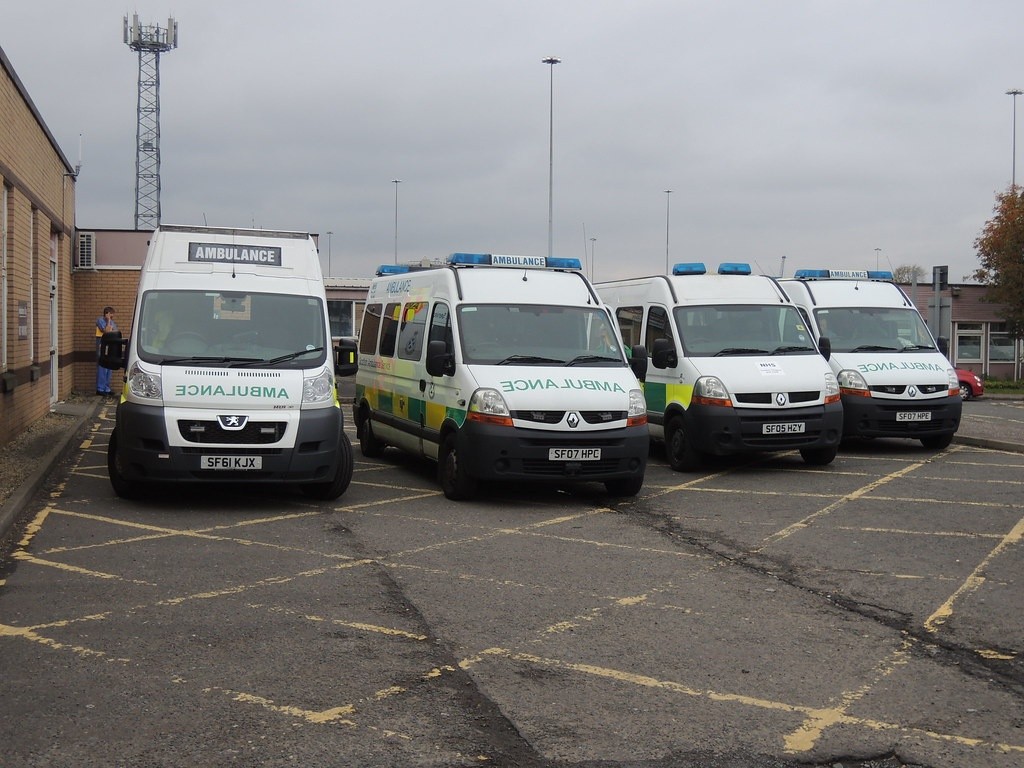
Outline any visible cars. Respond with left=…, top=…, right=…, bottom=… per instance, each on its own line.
left=954, top=367, right=985, bottom=401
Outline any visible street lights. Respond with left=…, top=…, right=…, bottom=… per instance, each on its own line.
left=663, top=188, right=674, bottom=277
left=391, top=179, right=403, bottom=262
left=327, top=231, right=333, bottom=278
left=1005, top=87, right=1023, bottom=192
left=590, top=237, right=596, bottom=284
left=542, top=56, right=562, bottom=258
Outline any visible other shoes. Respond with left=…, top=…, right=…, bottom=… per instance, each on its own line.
left=106, top=391, right=116, bottom=396
left=96, top=390, right=106, bottom=396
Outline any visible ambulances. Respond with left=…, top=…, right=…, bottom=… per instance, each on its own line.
left=99, top=224, right=362, bottom=503
left=588, top=261, right=845, bottom=479
left=776, top=266, right=964, bottom=453
left=354, top=253, right=650, bottom=507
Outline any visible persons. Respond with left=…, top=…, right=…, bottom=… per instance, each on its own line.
left=96, top=307, right=120, bottom=396
